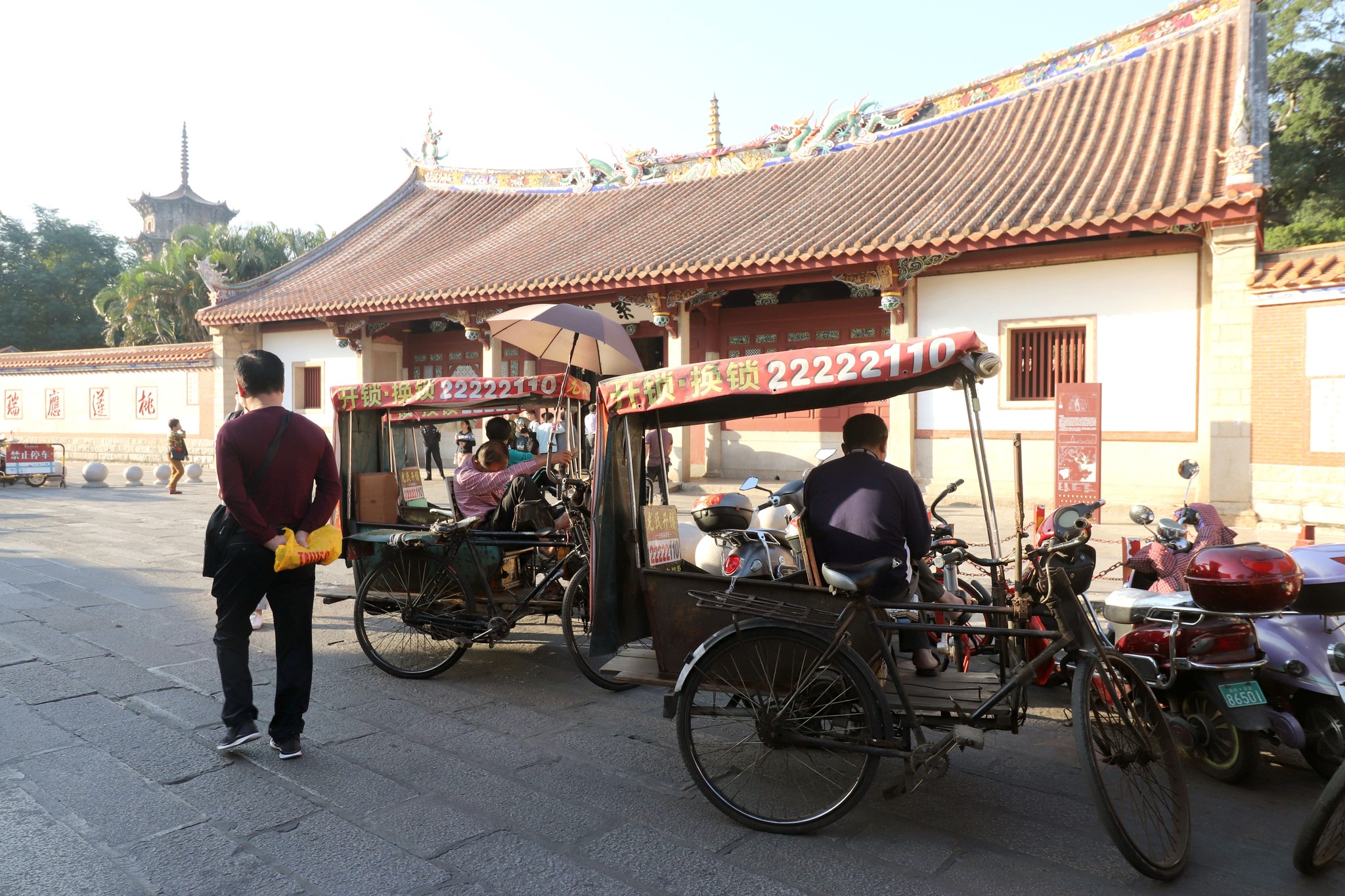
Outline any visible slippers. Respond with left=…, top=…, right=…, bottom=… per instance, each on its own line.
left=952, top=596, right=979, bottom=626
left=915, top=650, right=941, bottom=676
left=541, top=581, right=565, bottom=600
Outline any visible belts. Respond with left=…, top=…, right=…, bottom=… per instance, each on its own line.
left=586, top=434, right=595, bottom=435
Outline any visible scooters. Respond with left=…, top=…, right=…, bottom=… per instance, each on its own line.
left=690, top=447, right=837, bottom=585
left=1290, top=757, right=1345, bottom=875
left=0, top=438, right=49, bottom=488
left=1107, top=458, right=1345, bottom=782
left=999, top=458, right=1304, bottom=785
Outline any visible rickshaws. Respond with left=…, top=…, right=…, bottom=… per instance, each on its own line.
left=587, top=328, right=1194, bottom=883
left=329, top=371, right=667, bottom=692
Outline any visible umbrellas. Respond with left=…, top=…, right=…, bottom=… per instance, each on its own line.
left=486, top=304, right=645, bottom=446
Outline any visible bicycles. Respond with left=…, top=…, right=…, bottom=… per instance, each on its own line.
left=921, top=477, right=995, bottom=674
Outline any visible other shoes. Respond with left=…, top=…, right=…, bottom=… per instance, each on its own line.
left=166, top=486, right=182, bottom=494
left=249, top=611, right=263, bottom=629
left=442, top=475, right=445, bottom=480
left=270, top=737, right=302, bottom=759
left=217, top=719, right=263, bottom=750
left=424, top=477, right=432, bottom=480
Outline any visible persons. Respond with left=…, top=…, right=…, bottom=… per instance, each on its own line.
left=420, top=424, right=447, bottom=480
left=211, top=351, right=342, bottom=761
left=803, top=413, right=977, bottom=679
left=645, top=428, right=673, bottom=505
left=167, top=419, right=189, bottom=494
left=216, top=392, right=244, bottom=506
left=454, top=404, right=597, bottom=557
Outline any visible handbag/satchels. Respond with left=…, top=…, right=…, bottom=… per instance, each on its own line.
left=202, top=504, right=227, bottom=579
left=453, top=453, right=458, bottom=465
left=170, top=451, right=185, bottom=460
